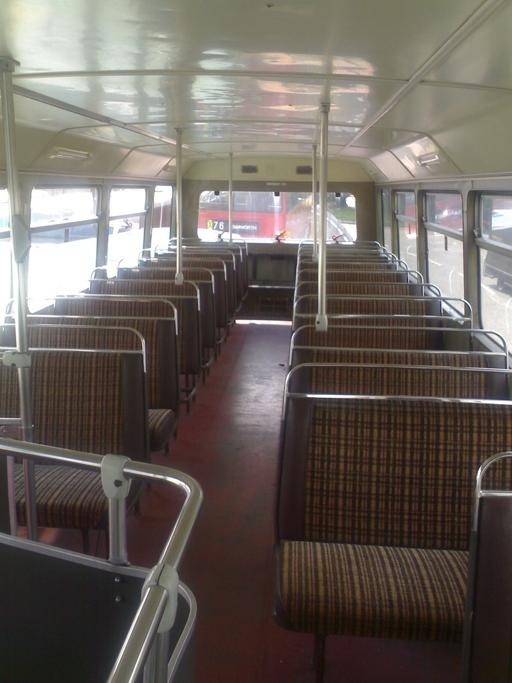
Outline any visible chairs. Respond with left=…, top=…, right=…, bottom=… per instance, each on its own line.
left=234, top=192, right=253, bottom=212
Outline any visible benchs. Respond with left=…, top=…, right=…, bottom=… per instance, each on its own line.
left=461, top=452, right=512, bottom=683
left=286, top=240, right=509, bottom=398
left=3, top=322, right=148, bottom=555
left=271, top=362, right=510, bottom=682
left=5, top=238, right=248, bottom=456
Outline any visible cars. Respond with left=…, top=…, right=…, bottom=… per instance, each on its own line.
left=142, top=182, right=316, bottom=243
